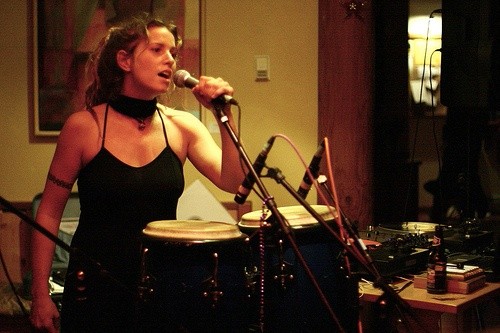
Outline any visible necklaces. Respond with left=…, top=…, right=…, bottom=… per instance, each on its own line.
left=136, top=119, right=145, bottom=129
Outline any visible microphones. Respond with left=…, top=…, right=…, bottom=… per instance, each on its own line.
left=298, top=140, right=326, bottom=199
left=234, top=135, right=276, bottom=205
left=173, top=69, right=238, bottom=105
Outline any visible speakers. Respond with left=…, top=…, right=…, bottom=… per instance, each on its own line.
left=440, top=0, right=500, bottom=113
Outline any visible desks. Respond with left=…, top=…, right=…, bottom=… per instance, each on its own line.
left=357, top=281, right=500, bottom=333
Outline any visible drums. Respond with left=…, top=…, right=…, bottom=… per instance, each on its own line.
left=138, top=219, right=249, bottom=333
left=236, top=203, right=362, bottom=333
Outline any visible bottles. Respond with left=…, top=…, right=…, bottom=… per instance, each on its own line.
left=426, top=226, right=446, bottom=295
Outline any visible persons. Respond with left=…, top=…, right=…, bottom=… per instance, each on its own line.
left=30, top=10, right=249, bottom=333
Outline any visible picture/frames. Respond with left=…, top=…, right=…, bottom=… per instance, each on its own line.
left=30, top=0, right=207, bottom=140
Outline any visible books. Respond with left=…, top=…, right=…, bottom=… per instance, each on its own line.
left=414, top=263, right=486, bottom=295
